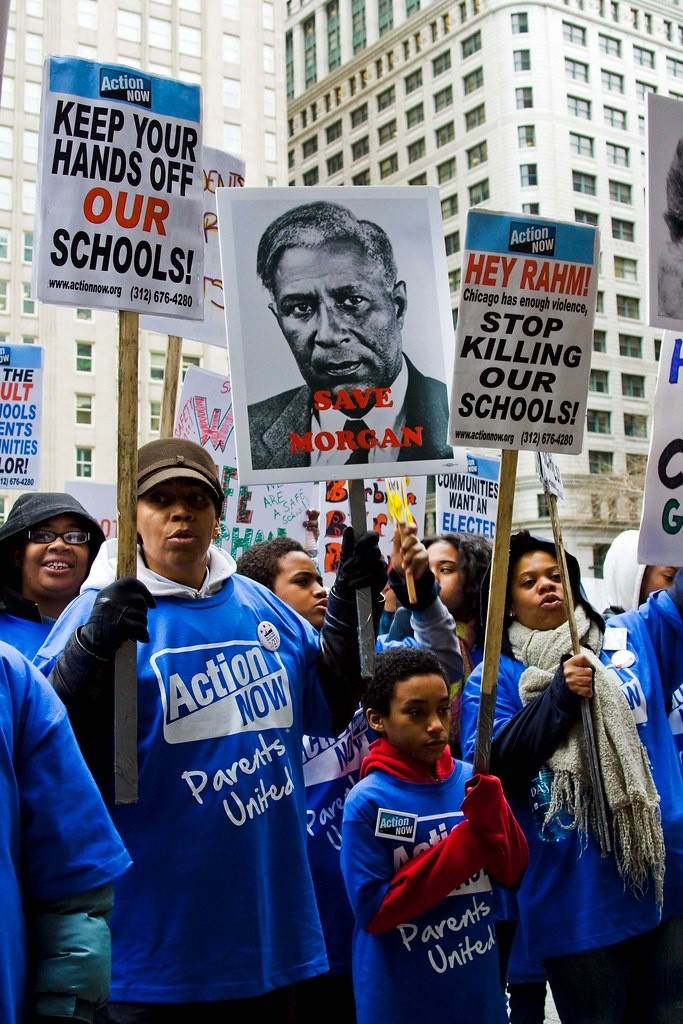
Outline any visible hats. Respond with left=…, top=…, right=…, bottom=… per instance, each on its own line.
left=137, top=438, right=225, bottom=517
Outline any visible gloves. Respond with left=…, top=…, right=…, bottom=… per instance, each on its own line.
left=80, top=577, right=157, bottom=657
left=334, top=527, right=389, bottom=597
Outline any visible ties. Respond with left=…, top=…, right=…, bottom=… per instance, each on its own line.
left=342, top=419, right=373, bottom=465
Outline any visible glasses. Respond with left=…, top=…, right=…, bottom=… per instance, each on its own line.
left=28, top=529, right=91, bottom=545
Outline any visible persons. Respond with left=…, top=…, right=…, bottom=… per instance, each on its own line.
left=0, top=438, right=683, bottom=1024
left=250, top=202, right=457, bottom=471
